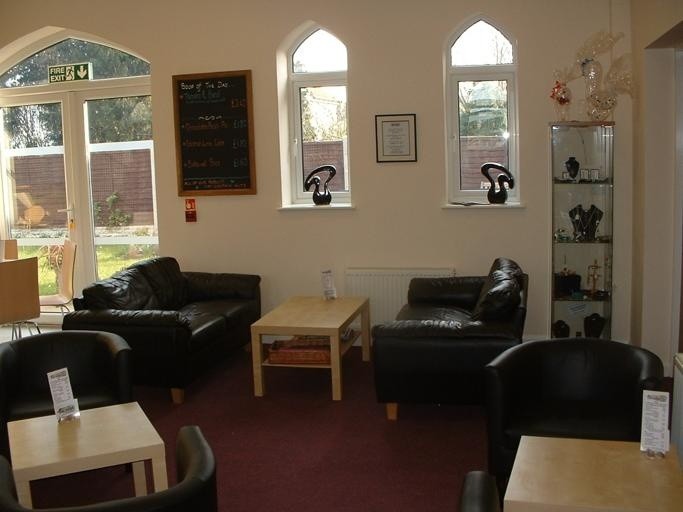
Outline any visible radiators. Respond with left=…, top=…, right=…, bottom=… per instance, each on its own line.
left=344, top=270, right=455, bottom=346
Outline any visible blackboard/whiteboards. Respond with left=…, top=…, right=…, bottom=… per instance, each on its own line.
left=171, top=69, right=257, bottom=197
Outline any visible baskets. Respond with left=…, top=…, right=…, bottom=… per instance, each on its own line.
left=268, top=338, right=331, bottom=365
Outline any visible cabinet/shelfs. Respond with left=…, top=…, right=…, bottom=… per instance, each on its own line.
left=550, top=121, right=614, bottom=339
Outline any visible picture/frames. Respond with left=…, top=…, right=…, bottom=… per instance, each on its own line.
left=375, top=114, right=417, bottom=163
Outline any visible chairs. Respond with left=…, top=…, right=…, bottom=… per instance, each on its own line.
left=457, top=471, right=501, bottom=511
left=483, top=337, right=664, bottom=477
left=0, top=240, right=77, bottom=340
left=0, top=425, right=217, bottom=512
left=0, top=330, right=134, bottom=473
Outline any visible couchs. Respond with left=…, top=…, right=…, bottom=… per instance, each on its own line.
left=62, top=257, right=261, bottom=404
left=371, top=257, right=529, bottom=421
